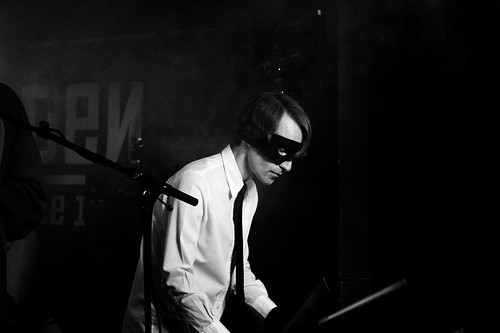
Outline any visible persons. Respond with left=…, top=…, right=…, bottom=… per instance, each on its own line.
left=120, top=89, right=312, bottom=333
left=0, top=82, right=47, bottom=333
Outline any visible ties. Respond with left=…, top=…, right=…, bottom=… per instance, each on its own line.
left=226, top=183, right=248, bottom=331
